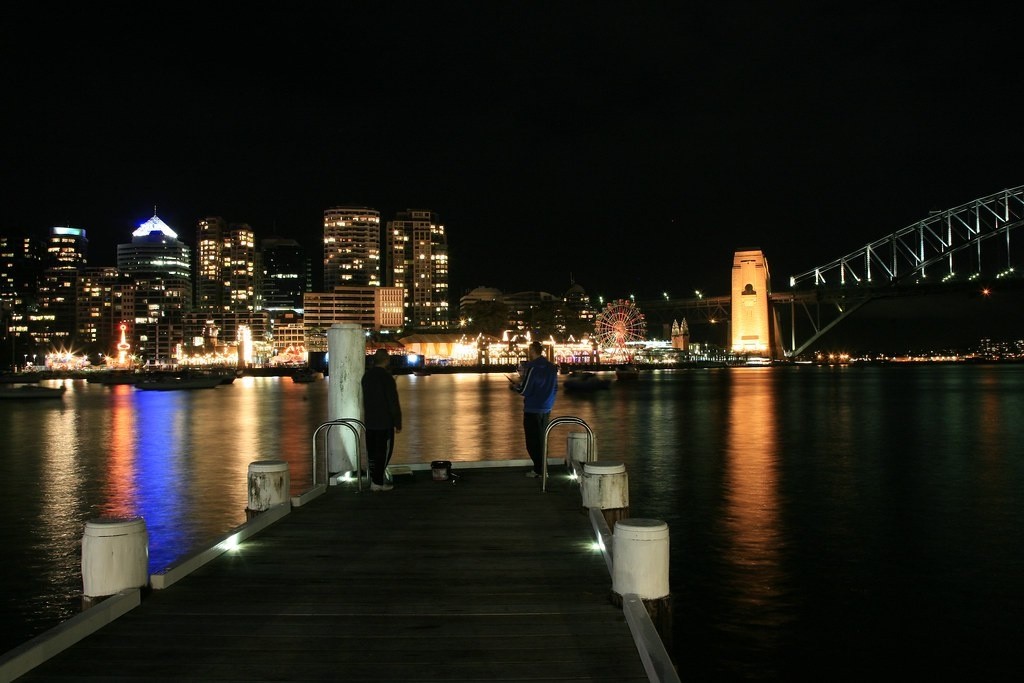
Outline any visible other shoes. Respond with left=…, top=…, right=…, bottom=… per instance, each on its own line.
left=525, top=470, right=549, bottom=477
left=370, top=481, right=393, bottom=490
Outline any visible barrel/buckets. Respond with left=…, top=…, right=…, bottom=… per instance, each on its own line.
left=613, top=518, right=671, bottom=599
left=247, top=460, right=290, bottom=512
left=582, top=461, right=629, bottom=509
left=568, top=432, right=597, bottom=467
left=81, top=517, right=148, bottom=597
left=432, top=460, right=451, bottom=481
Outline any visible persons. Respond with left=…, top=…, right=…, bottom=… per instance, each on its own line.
left=509, top=341, right=558, bottom=478
left=361, top=349, right=402, bottom=490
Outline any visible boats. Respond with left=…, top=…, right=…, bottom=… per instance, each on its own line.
left=86, top=365, right=237, bottom=391
left=0, top=383, right=67, bottom=401
left=615, top=364, right=640, bottom=381
left=563, top=370, right=611, bottom=391
left=292, top=366, right=325, bottom=382
